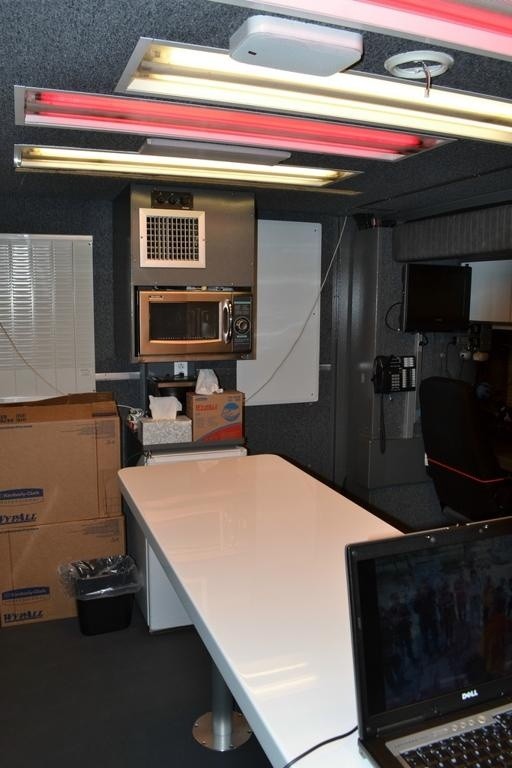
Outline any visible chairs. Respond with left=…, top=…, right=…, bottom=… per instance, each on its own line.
left=419, top=377, right=512, bottom=524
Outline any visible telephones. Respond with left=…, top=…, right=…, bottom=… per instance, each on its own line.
left=373, top=355, right=417, bottom=394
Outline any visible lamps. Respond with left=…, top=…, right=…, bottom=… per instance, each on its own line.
left=9, top=1, right=511, bottom=197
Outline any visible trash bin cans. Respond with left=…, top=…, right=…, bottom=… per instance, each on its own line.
left=69, top=554, right=137, bottom=636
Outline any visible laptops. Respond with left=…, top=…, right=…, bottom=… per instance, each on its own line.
left=345, top=518, right=512, bottom=768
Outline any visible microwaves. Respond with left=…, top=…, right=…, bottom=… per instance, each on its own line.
left=140, top=291, right=252, bottom=355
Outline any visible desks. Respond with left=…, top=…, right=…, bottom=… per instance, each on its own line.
left=111, top=450, right=511, bottom=767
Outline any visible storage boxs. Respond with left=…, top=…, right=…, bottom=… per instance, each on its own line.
left=187, top=392, right=249, bottom=445
left=1, top=397, right=131, bottom=635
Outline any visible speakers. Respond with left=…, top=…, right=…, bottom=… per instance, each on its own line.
left=403, top=263, right=472, bottom=332
